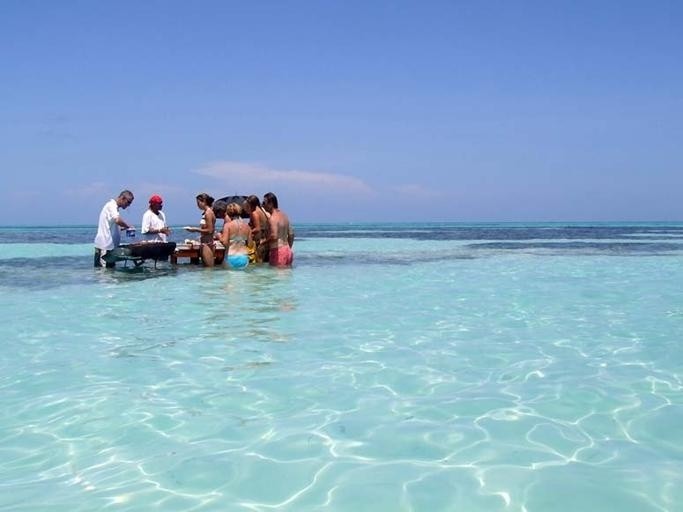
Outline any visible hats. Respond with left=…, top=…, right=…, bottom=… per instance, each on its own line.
left=149, top=194, right=163, bottom=204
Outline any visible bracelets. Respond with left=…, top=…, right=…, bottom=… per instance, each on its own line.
left=158, top=229, right=159, bottom=233
left=195, top=228, right=198, bottom=231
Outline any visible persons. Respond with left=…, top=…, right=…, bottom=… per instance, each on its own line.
left=211, top=192, right=295, bottom=270
left=140, top=195, right=171, bottom=260
left=92, top=190, right=133, bottom=268
left=183, top=193, right=216, bottom=268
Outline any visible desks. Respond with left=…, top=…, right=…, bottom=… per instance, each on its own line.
left=110, top=242, right=177, bottom=270
left=170, top=242, right=225, bottom=265
